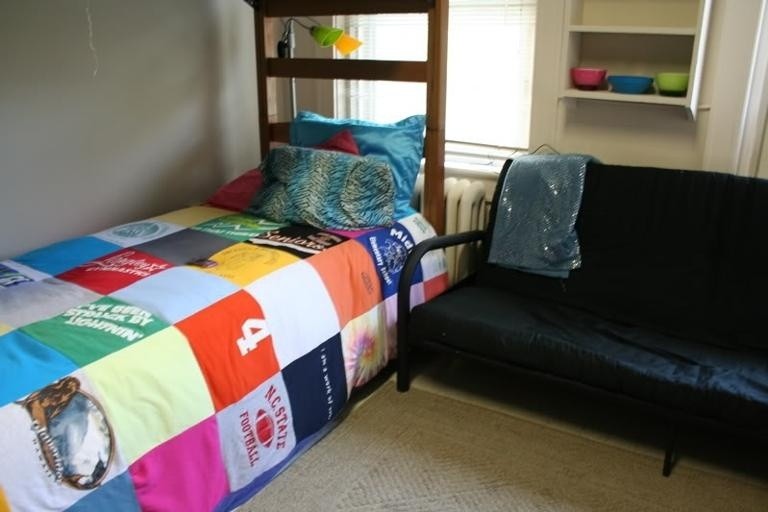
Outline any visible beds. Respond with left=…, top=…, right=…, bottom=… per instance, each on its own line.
left=0, top=203, right=450, bottom=512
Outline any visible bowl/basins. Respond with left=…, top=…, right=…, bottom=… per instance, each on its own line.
left=606, top=75, right=653, bottom=95
left=657, top=72, right=688, bottom=97
left=571, top=67, right=607, bottom=90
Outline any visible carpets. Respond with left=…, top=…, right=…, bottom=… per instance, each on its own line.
left=232, top=380, right=768, bottom=512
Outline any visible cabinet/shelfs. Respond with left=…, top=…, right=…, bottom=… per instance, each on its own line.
left=557, top=1, right=712, bottom=121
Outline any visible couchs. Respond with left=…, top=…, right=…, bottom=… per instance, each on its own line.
left=397, top=158, right=768, bottom=481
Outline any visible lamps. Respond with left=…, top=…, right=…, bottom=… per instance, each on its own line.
left=277, top=16, right=364, bottom=121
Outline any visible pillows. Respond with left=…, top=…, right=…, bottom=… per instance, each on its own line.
left=289, top=109, right=428, bottom=217
left=241, top=146, right=395, bottom=230
left=205, top=129, right=359, bottom=213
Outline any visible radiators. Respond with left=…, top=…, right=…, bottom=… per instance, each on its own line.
left=412, top=171, right=486, bottom=288
